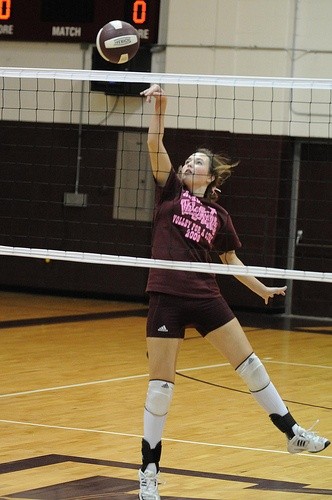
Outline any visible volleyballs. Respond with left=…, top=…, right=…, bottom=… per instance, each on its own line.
left=97, top=20, right=140, bottom=64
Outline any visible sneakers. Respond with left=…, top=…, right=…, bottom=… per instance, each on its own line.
left=287, top=419, right=331, bottom=454
left=138, top=462, right=160, bottom=500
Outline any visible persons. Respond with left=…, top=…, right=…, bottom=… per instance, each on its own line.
left=137, top=84, right=331, bottom=500
